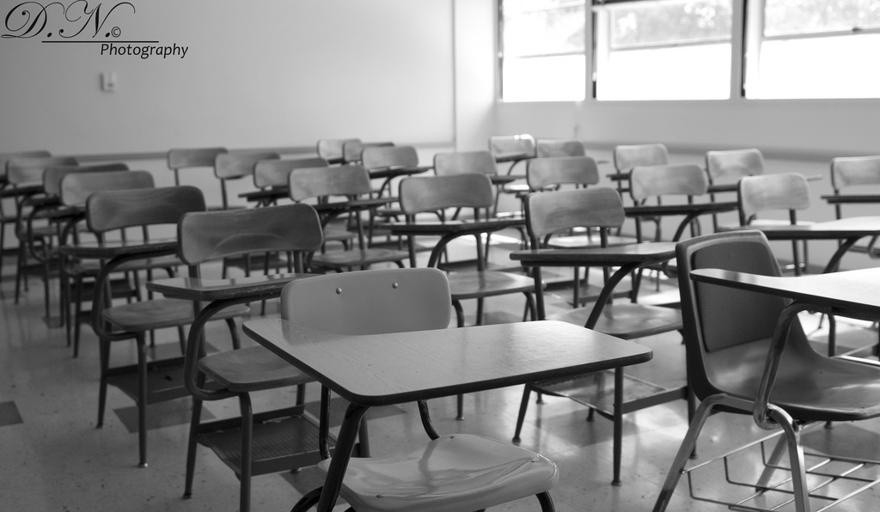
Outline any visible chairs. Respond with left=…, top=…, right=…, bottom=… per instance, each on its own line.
left=0, top=138, right=880, bottom=512
